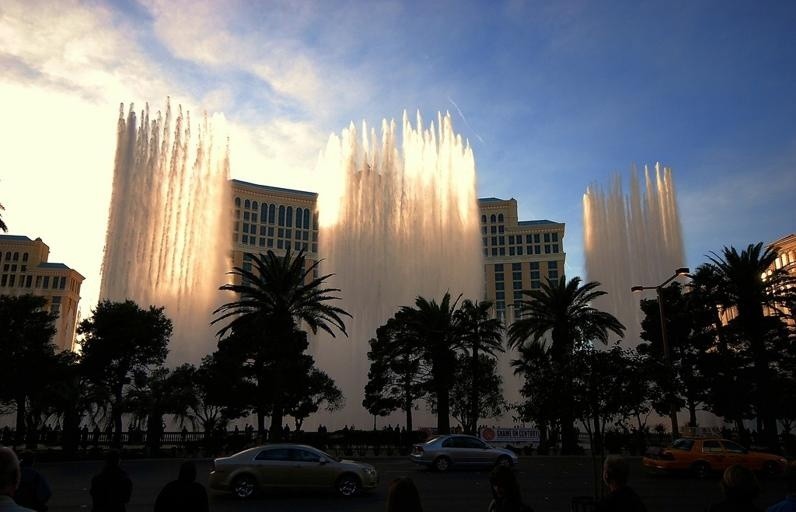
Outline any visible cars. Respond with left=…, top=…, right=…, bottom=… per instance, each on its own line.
left=641, top=433, right=789, bottom=485
left=203, top=438, right=381, bottom=503
left=406, top=433, right=520, bottom=474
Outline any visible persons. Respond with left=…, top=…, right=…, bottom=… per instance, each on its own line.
left=0, top=422, right=796, bottom=512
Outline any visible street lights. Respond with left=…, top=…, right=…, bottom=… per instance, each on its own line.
left=630, top=265, right=692, bottom=443
